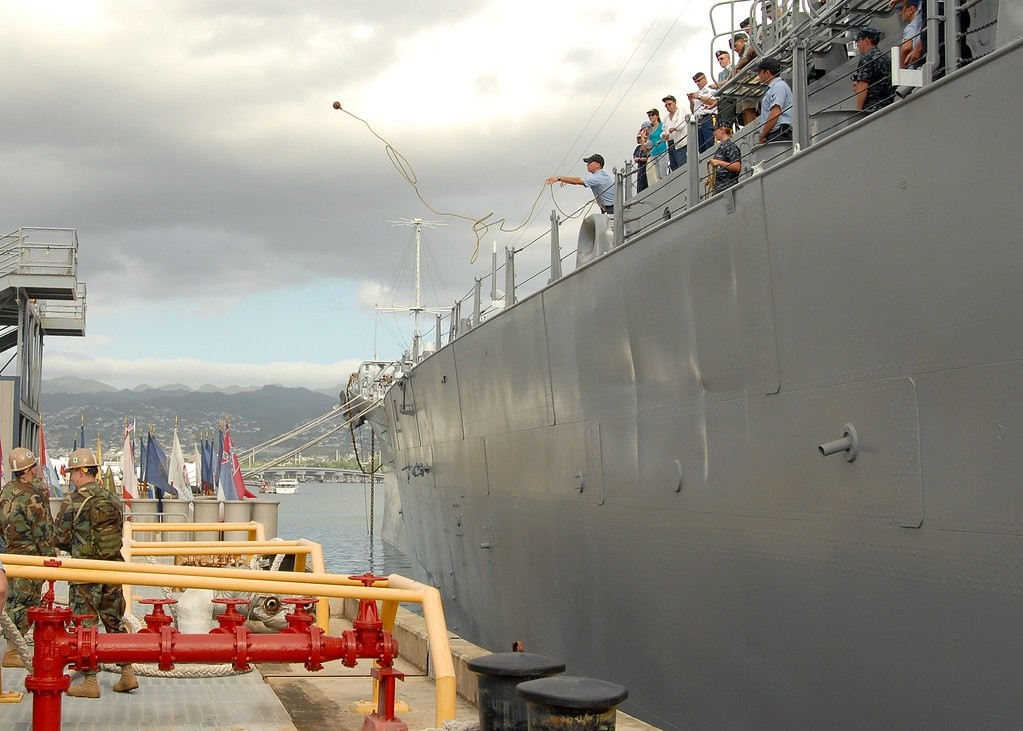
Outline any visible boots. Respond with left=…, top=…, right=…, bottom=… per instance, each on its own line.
left=66, top=674, right=100, bottom=698
left=2, top=640, right=28, bottom=667
left=112, top=664, right=139, bottom=692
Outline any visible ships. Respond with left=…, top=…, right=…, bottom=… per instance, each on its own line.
left=328, top=0, right=1023, bottom=731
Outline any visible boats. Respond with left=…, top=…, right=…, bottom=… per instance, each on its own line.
left=274, top=478, right=300, bottom=494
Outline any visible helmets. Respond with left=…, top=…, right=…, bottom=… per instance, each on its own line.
left=63, top=449, right=101, bottom=472
left=9, top=447, right=39, bottom=471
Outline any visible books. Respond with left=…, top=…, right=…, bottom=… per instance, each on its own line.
left=687, top=0, right=793, bottom=154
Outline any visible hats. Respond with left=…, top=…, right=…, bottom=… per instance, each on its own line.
left=583, top=153, right=604, bottom=166
left=716, top=16, right=756, bottom=57
left=849, top=26, right=881, bottom=40
left=895, top=0, right=919, bottom=9
left=692, top=72, right=703, bottom=80
left=752, top=57, right=781, bottom=71
left=708, top=118, right=733, bottom=131
left=636, top=95, right=676, bottom=144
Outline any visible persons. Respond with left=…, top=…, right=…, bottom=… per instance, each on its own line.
left=0, top=562, right=8, bottom=615
left=632, top=95, right=688, bottom=195
left=751, top=56, right=793, bottom=143
left=703, top=119, right=741, bottom=196
left=0, top=447, right=59, bottom=669
left=55, top=449, right=140, bottom=699
left=547, top=154, right=614, bottom=214
left=850, top=0, right=973, bottom=117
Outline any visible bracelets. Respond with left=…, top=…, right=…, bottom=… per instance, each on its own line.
left=697, top=94, right=702, bottom=100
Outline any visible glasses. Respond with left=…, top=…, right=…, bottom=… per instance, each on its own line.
left=718, top=57, right=725, bottom=60
left=648, top=112, right=656, bottom=117
left=665, top=101, right=674, bottom=107
left=695, top=78, right=703, bottom=83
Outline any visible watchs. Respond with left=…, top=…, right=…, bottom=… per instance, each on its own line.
left=760, top=131, right=765, bottom=136
left=558, top=176, right=561, bottom=181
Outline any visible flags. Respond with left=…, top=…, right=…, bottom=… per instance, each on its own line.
left=0, top=439, right=6, bottom=492
left=123, top=428, right=257, bottom=522
left=42, top=428, right=85, bottom=497
